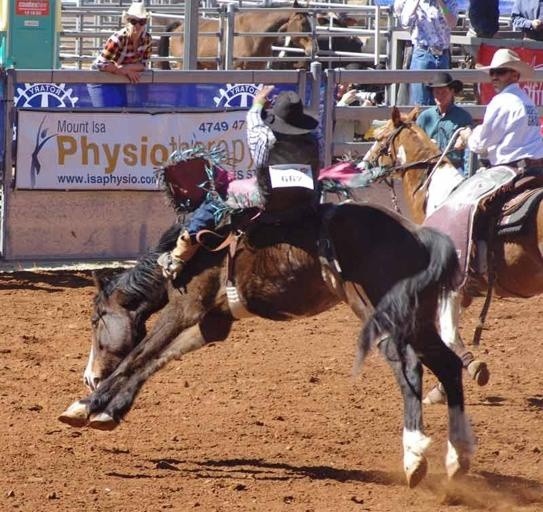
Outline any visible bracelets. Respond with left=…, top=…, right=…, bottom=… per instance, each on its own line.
left=439, top=7, right=448, bottom=14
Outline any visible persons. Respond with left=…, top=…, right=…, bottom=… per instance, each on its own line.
left=86, top=2, right=153, bottom=108
left=457, top=47, right=543, bottom=289
left=510, top=0, right=542, bottom=39
left=466, top=0, right=499, bottom=38
left=414, top=71, right=472, bottom=171
left=332, top=67, right=372, bottom=107
left=400, top=0, right=459, bottom=105
left=156, top=85, right=321, bottom=282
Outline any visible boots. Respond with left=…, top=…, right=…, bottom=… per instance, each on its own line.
left=157, top=230, right=200, bottom=280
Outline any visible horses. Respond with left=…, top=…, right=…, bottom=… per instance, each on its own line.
left=156, top=0, right=319, bottom=71
left=352, top=102, right=543, bottom=420
left=269, top=13, right=363, bottom=71
left=55, top=200, right=473, bottom=490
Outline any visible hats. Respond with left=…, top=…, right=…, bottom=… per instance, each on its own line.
left=475, top=48, right=535, bottom=79
left=424, top=72, right=463, bottom=93
left=121, top=2, right=150, bottom=24
left=261, top=90, right=319, bottom=136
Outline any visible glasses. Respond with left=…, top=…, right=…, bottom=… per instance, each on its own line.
left=489, top=69, right=515, bottom=76
left=129, top=18, right=147, bottom=25
